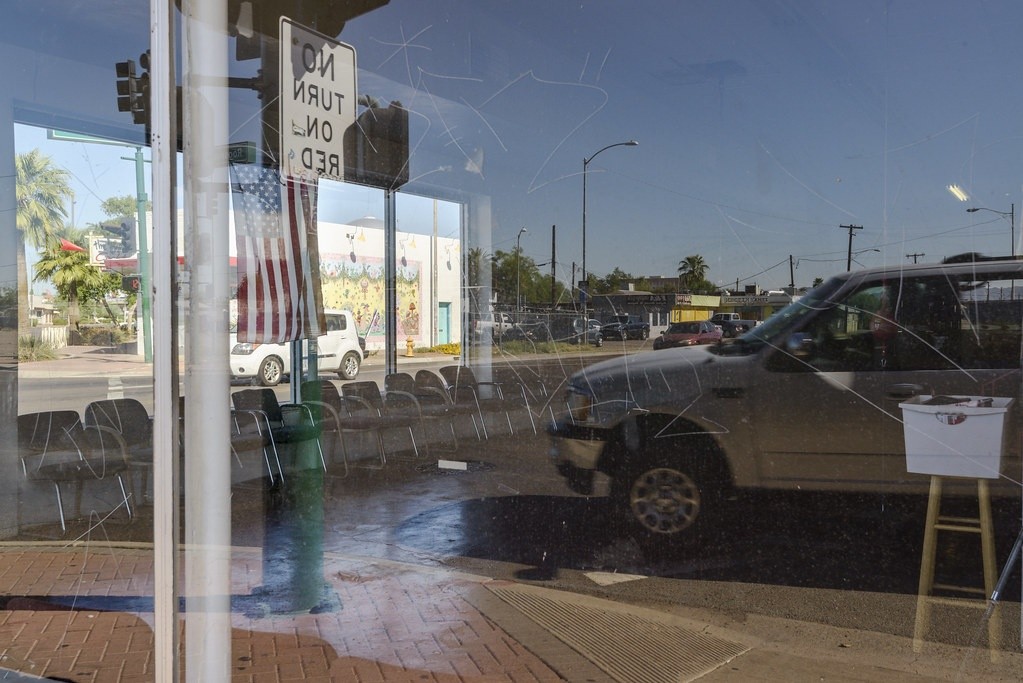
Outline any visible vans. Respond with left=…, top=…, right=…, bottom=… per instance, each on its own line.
left=466, top=310, right=519, bottom=338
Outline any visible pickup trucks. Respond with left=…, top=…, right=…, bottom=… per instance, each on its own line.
left=707, top=312, right=757, bottom=338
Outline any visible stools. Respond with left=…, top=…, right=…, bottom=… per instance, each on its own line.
left=913, top=474, right=1004, bottom=663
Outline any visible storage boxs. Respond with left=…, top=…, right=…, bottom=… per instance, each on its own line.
left=898, top=394, right=1015, bottom=479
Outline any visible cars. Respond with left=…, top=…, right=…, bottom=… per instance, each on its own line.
left=652, top=321, right=722, bottom=350
left=561, top=316, right=650, bottom=347
left=503, top=319, right=551, bottom=341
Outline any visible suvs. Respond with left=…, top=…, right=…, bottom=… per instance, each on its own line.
left=535, top=256, right=1023, bottom=554
left=229, top=308, right=365, bottom=387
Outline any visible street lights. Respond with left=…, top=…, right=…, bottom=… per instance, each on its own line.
left=515, top=226, right=528, bottom=329
left=967, top=204, right=1015, bottom=302
left=844, top=248, right=881, bottom=333
left=582, top=140, right=639, bottom=346
left=383, top=163, right=454, bottom=379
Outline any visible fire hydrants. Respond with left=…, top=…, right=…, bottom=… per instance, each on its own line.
left=406, top=335, right=416, bottom=360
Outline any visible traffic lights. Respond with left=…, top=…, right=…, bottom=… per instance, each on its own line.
left=120, top=217, right=138, bottom=257
left=139, top=47, right=152, bottom=103
left=116, top=60, right=136, bottom=112
left=131, top=78, right=148, bottom=126
left=122, top=276, right=141, bottom=291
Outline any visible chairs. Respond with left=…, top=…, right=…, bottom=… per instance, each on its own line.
left=18, top=366, right=573, bottom=539
left=327, top=320, right=336, bottom=330
